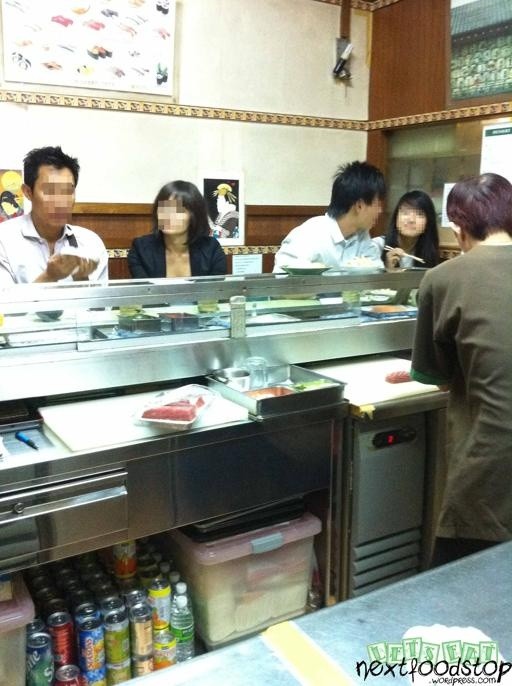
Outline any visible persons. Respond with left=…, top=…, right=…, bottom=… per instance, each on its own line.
left=0, top=145, right=111, bottom=286
left=406, top=172, right=511, bottom=569
left=126, top=180, right=228, bottom=307
left=271, top=160, right=388, bottom=298
left=0, top=191, right=24, bottom=222
left=373, top=189, right=440, bottom=269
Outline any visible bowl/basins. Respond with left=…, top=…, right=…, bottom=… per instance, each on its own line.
left=36, top=310, right=63, bottom=322
left=212, top=368, right=249, bottom=393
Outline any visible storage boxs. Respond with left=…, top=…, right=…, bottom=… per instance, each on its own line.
left=151, top=511, right=329, bottom=655
left=1, top=568, right=44, bottom=686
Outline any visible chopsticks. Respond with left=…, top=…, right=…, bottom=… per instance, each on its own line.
left=381, top=245, right=425, bottom=264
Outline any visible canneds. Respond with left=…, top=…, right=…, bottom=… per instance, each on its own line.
left=26, top=540, right=176, bottom=686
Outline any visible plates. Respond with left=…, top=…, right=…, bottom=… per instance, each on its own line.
left=279, top=263, right=332, bottom=275
left=245, top=388, right=295, bottom=401
left=339, top=264, right=380, bottom=275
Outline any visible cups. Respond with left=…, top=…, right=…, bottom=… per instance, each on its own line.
left=245, top=356, right=267, bottom=389
left=119, top=303, right=144, bottom=317
left=197, top=298, right=219, bottom=314
left=342, top=290, right=360, bottom=305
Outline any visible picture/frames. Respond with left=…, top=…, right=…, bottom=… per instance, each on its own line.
left=0, top=162, right=33, bottom=221
left=1, top=0, right=182, bottom=105
left=193, top=171, right=246, bottom=247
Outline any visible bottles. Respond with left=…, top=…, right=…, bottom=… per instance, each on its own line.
left=150, top=549, right=194, bottom=668
